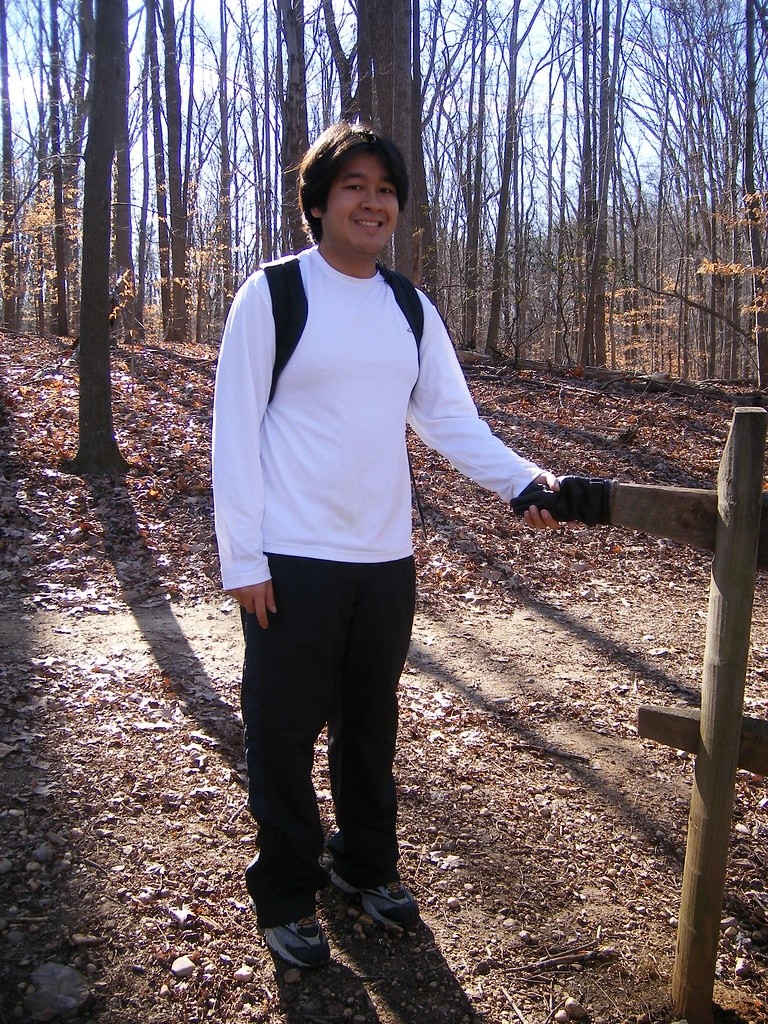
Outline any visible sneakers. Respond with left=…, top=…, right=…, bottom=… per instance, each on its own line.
left=329, top=865, right=419, bottom=926
left=248, top=895, right=330, bottom=968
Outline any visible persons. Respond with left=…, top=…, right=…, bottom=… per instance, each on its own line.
left=213, top=122, right=561, bottom=972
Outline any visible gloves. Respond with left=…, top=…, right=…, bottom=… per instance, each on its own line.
left=510, top=476, right=612, bottom=527
